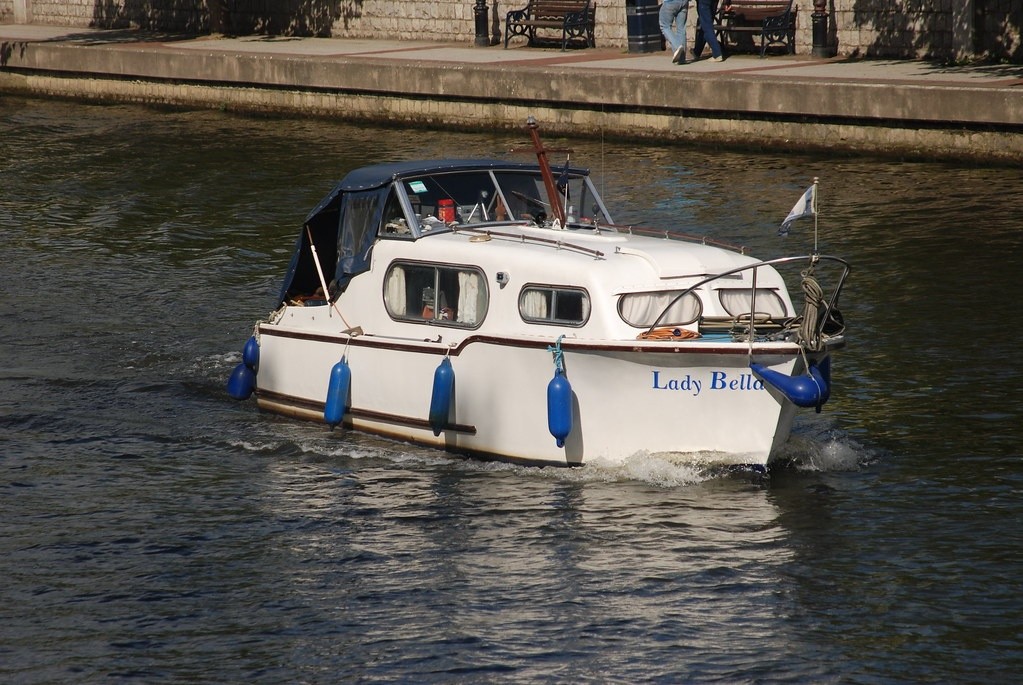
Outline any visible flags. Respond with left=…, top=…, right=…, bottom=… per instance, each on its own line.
left=778, top=184, right=816, bottom=238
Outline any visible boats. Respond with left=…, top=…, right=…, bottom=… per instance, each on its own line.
left=253, top=117, right=849, bottom=479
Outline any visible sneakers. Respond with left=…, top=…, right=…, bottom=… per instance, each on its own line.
left=671, top=44, right=687, bottom=65
left=688, top=47, right=699, bottom=59
left=708, top=54, right=723, bottom=61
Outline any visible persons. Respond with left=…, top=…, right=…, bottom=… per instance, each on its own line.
left=689, top=0, right=725, bottom=62
left=658, top=0, right=689, bottom=65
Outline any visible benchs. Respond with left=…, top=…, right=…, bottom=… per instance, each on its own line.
left=504, top=0, right=597, bottom=52
left=695, top=0, right=798, bottom=59
